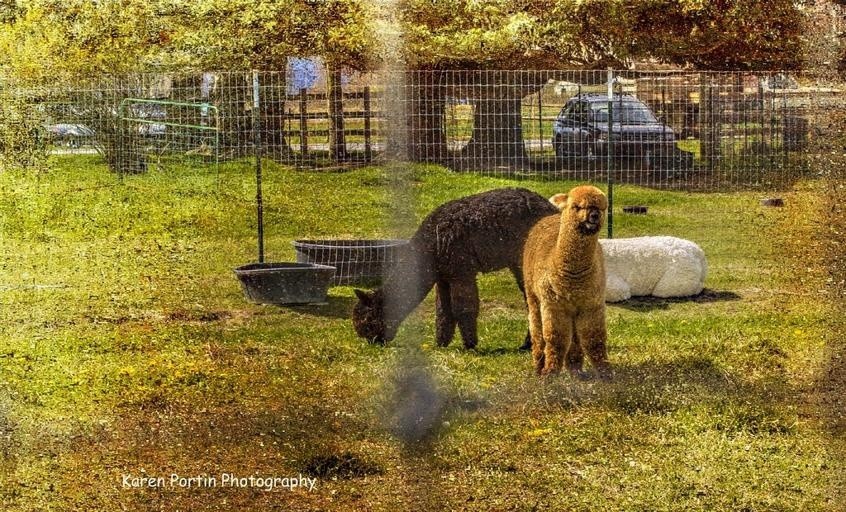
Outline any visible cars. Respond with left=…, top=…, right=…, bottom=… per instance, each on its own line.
left=49, top=124, right=97, bottom=148
left=552, top=92, right=676, bottom=163
